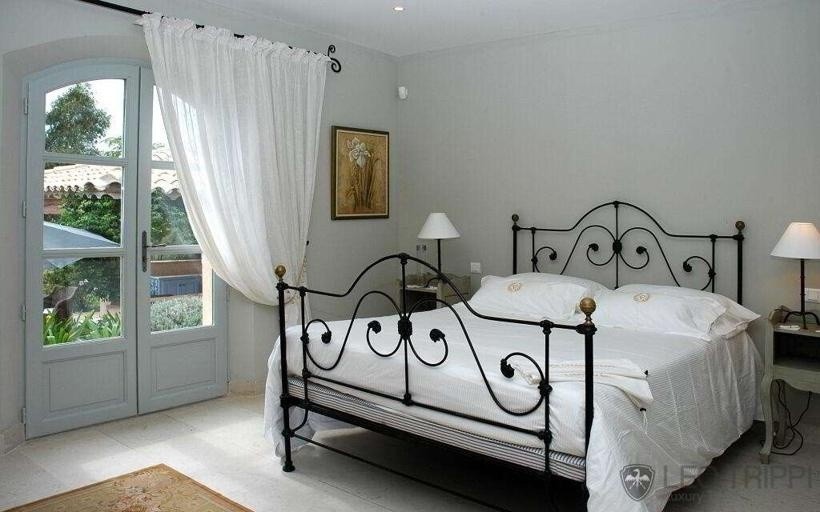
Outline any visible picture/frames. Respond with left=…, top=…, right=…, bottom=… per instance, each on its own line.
left=331, top=126, right=390, bottom=220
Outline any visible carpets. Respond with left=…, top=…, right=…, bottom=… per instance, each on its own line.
left=0, top=465, right=254, bottom=512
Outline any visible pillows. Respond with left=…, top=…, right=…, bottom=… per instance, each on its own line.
left=467, top=273, right=615, bottom=327
left=593, top=283, right=762, bottom=344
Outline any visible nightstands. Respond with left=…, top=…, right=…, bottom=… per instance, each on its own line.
left=394, top=273, right=470, bottom=314
left=758, top=307, right=820, bottom=465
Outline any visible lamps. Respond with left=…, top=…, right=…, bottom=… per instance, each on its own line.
left=417, top=212, right=461, bottom=285
left=769, top=222, right=820, bottom=330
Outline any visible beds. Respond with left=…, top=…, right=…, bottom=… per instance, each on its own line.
left=275, top=201, right=748, bottom=512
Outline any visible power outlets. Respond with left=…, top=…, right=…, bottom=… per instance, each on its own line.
left=804, top=287, right=820, bottom=304
left=470, top=261, right=482, bottom=275
